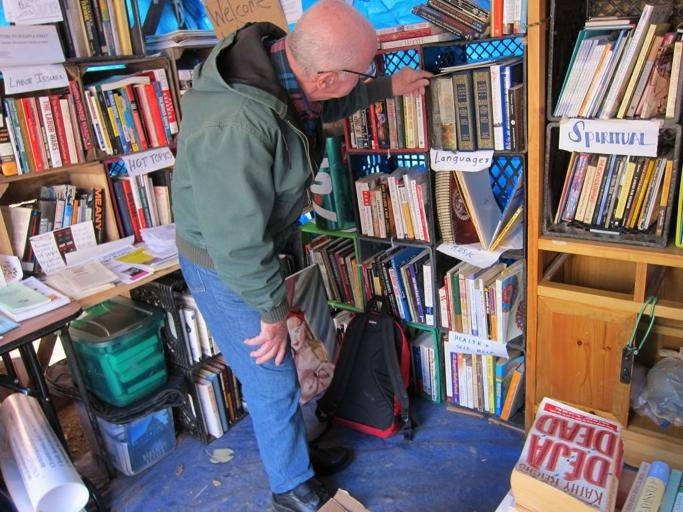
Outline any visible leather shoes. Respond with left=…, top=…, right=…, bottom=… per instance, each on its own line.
left=309, top=443, right=352, bottom=476
left=272, top=478, right=330, bottom=512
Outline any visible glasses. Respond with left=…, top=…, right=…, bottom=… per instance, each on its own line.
left=317, top=60, right=378, bottom=84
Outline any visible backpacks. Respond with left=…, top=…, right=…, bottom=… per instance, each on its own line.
left=315, top=297, right=414, bottom=440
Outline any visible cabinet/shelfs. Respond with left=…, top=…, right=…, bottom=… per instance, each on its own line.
left=0, top=1, right=203, bottom=510
left=203, top=5, right=525, bottom=443
left=526, top=2, right=683, bottom=477
